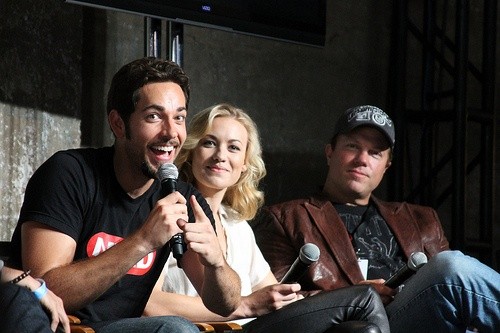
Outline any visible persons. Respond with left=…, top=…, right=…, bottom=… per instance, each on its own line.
left=8, top=57, right=242, bottom=333
left=253, top=104, right=500, bottom=333
left=141, top=104, right=390, bottom=333
left=0, top=259, right=71, bottom=333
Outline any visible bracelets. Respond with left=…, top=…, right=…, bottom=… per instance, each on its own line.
left=31, top=277, right=48, bottom=299
left=6, top=269, right=31, bottom=285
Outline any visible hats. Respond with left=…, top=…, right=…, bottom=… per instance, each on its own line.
left=334, top=105, right=395, bottom=152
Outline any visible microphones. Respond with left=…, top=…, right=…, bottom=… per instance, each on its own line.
left=158, top=162, right=185, bottom=259
left=383, top=251, right=428, bottom=289
left=280, top=243, right=321, bottom=285
